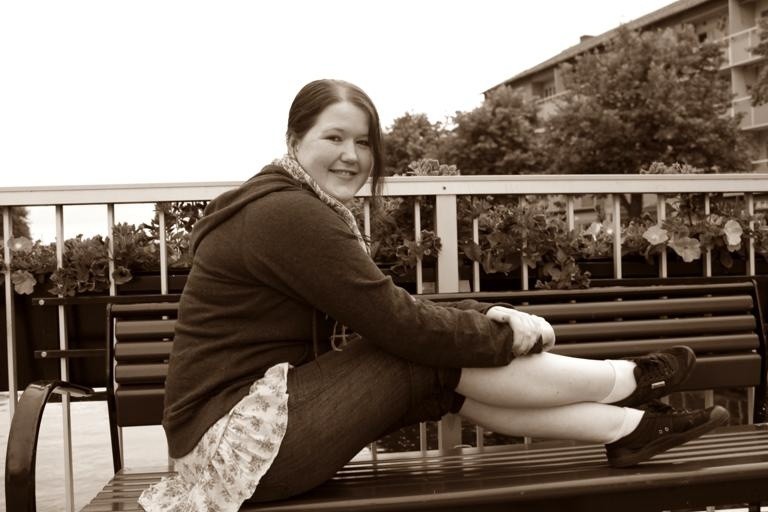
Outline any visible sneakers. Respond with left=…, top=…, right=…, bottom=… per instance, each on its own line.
left=607, top=345, right=696, bottom=406
left=605, top=406, right=729, bottom=468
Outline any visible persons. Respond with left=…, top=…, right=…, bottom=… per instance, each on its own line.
left=139, top=78, right=730, bottom=512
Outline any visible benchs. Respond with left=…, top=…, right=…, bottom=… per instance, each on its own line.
left=2, top=271, right=768, bottom=512
